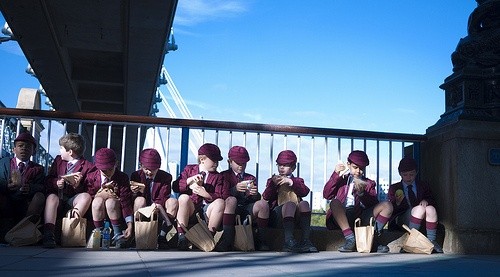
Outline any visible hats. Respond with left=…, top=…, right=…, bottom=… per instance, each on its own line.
left=347, top=150, right=369, bottom=166
left=13, top=132, right=38, bottom=147
left=198, top=143, right=297, bottom=166
left=398, top=156, right=418, bottom=170
left=95, top=148, right=161, bottom=170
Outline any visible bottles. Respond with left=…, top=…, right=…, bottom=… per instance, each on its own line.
left=93, top=229, right=100, bottom=248
left=102, top=224, right=110, bottom=248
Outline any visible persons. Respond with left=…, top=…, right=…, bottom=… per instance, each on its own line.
left=262, top=150, right=318, bottom=252
left=130, top=149, right=172, bottom=233
left=83, top=148, right=133, bottom=245
left=387, top=156, right=443, bottom=253
left=216, top=146, right=272, bottom=251
left=43, top=133, right=96, bottom=248
left=172, top=143, right=225, bottom=251
left=323, top=150, right=394, bottom=252
left=0, top=133, right=45, bottom=224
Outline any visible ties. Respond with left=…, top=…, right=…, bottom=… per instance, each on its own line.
left=197, top=171, right=206, bottom=212
left=68, top=163, right=73, bottom=169
left=354, top=192, right=361, bottom=219
left=407, top=185, right=418, bottom=207
left=237, top=174, right=243, bottom=181
left=18, top=162, right=25, bottom=174
left=105, top=177, right=109, bottom=183
left=146, top=178, right=153, bottom=206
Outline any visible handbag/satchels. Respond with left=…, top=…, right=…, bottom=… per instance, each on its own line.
left=398, top=225, right=434, bottom=254
left=135, top=210, right=158, bottom=248
left=5, top=208, right=86, bottom=248
left=234, top=215, right=255, bottom=251
left=278, top=176, right=300, bottom=206
left=354, top=217, right=375, bottom=253
left=182, top=213, right=218, bottom=251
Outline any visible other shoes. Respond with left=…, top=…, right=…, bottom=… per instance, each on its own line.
left=115, top=240, right=130, bottom=248
left=216, top=238, right=233, bottom=252
left=256, top=237, right=271, bottom=250
left=284, top=239, right=318, bottom=253
left=372, top=243, right=389, bottom=253
left=338, top=238, right=356, bottom=252
left=431, top=241, right=444, bottom=253
left=158, top=236, right=189, bottom=251
